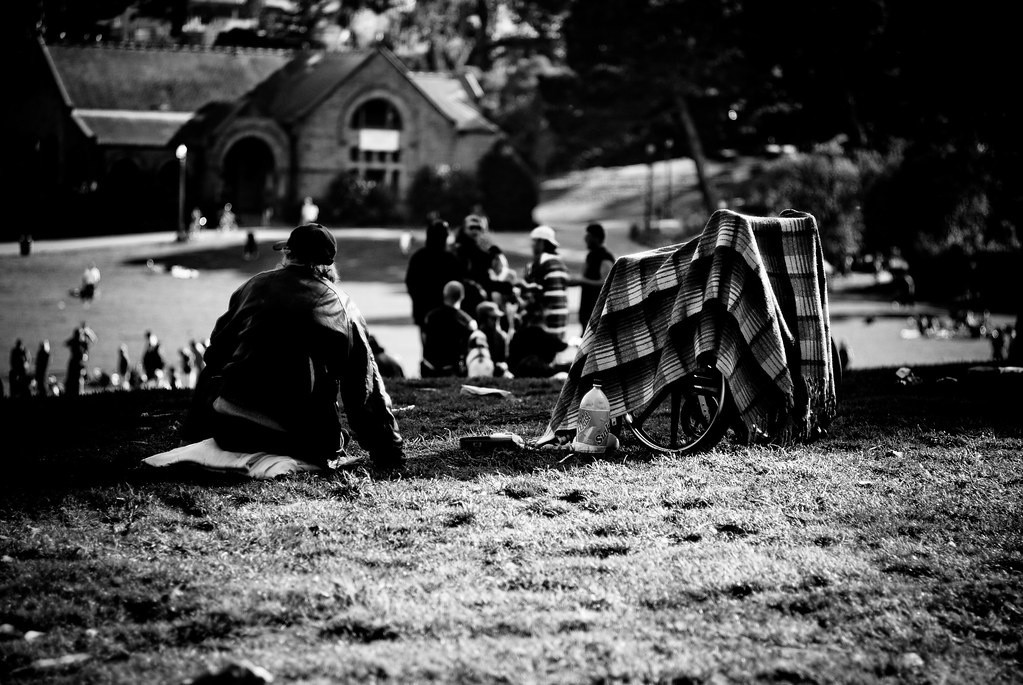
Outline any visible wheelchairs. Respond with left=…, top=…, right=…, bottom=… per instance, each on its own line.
left=624, top=358, right=827, bottom=456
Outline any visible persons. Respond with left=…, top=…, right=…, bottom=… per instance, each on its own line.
left=985, top=326, right=1016, bottom=359
left=9, top=322, right=211, bottom=390
left=300, top=197, right=319, bottom=224
left=404, top=214, right=615, bottom=379
left=179, top=225, right=406, bottom=470
left=80, top=261, right=102, bottom=310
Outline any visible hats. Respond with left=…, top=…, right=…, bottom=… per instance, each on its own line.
left=273, top=224, right=337, bottom=264
left=530, top=226, right=560, bottom=248
left=477, top=301, right=505, bottom=319
left=464, top=215, right=483, bottom=231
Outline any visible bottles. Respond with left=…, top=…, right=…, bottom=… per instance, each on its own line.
left=575, top=379, right=610, bottom=454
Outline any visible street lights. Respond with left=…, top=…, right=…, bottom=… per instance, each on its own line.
left=176, top=144, right=190, bottom=242
